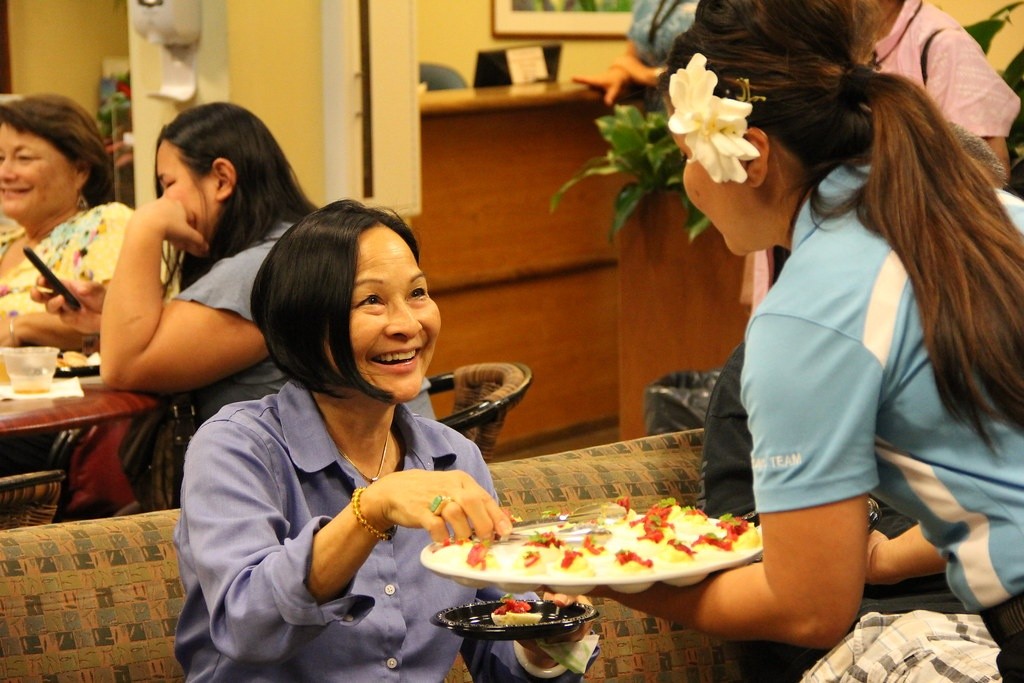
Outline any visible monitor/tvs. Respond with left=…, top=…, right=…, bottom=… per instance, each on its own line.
left=474, top=44, right=562, bottom=87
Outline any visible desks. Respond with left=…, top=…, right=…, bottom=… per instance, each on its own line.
left=0, top=382, right=159, bottom=536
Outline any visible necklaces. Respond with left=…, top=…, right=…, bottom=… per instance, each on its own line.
left=335, top=430, right=389, bottom=482
left=648, top=0, right=680, bottom=44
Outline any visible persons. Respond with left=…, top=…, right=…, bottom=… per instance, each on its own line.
left=862, top=0, right=1020, bottom=193
left=171, top=197, right=601, bottom=683
left=701, top=245, right=1003, bottom=683
left=31, top=103, right=438, bottom=422
left=0, top=94, right=166, bottom=353
left=537, top=0, right=1023, bottom=683
left=572, top=0, right=701, bottom=116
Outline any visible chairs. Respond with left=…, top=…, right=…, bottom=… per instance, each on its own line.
left=426, top=360, right=532, bottom=462
left=0, top=470, right=66, bottom=531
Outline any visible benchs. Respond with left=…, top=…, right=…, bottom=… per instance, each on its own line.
left=0, top=424, right=755, bottom=683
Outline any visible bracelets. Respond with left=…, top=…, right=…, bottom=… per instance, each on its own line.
left=10, top=317, right=16, bottom=347
left=351, top=486, right=398, bottom=539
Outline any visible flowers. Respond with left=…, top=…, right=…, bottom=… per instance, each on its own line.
left=663, top=54, right=768, bottom=188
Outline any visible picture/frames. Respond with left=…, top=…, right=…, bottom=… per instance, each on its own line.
left=490, top=0, right=642, bottom=40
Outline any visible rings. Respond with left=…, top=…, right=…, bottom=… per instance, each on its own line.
left=431, top=495, right=455, bottom=515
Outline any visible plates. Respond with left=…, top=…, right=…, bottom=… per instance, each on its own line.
left=429, top=600, right=600, bottom=641
left=738, top=494, right=883, bottom=534
left=53, top=354, right=101, bottom=379
left=418, top=514, right=764, bottom=596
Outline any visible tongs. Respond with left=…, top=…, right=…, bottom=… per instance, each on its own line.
left=465, top=502, right=626, bottom=543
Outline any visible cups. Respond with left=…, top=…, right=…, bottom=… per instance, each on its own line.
left=2, top=346, right=61, bottom=394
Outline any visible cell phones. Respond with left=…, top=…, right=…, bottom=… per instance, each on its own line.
left=24, top=247, right=81, bottom=311
